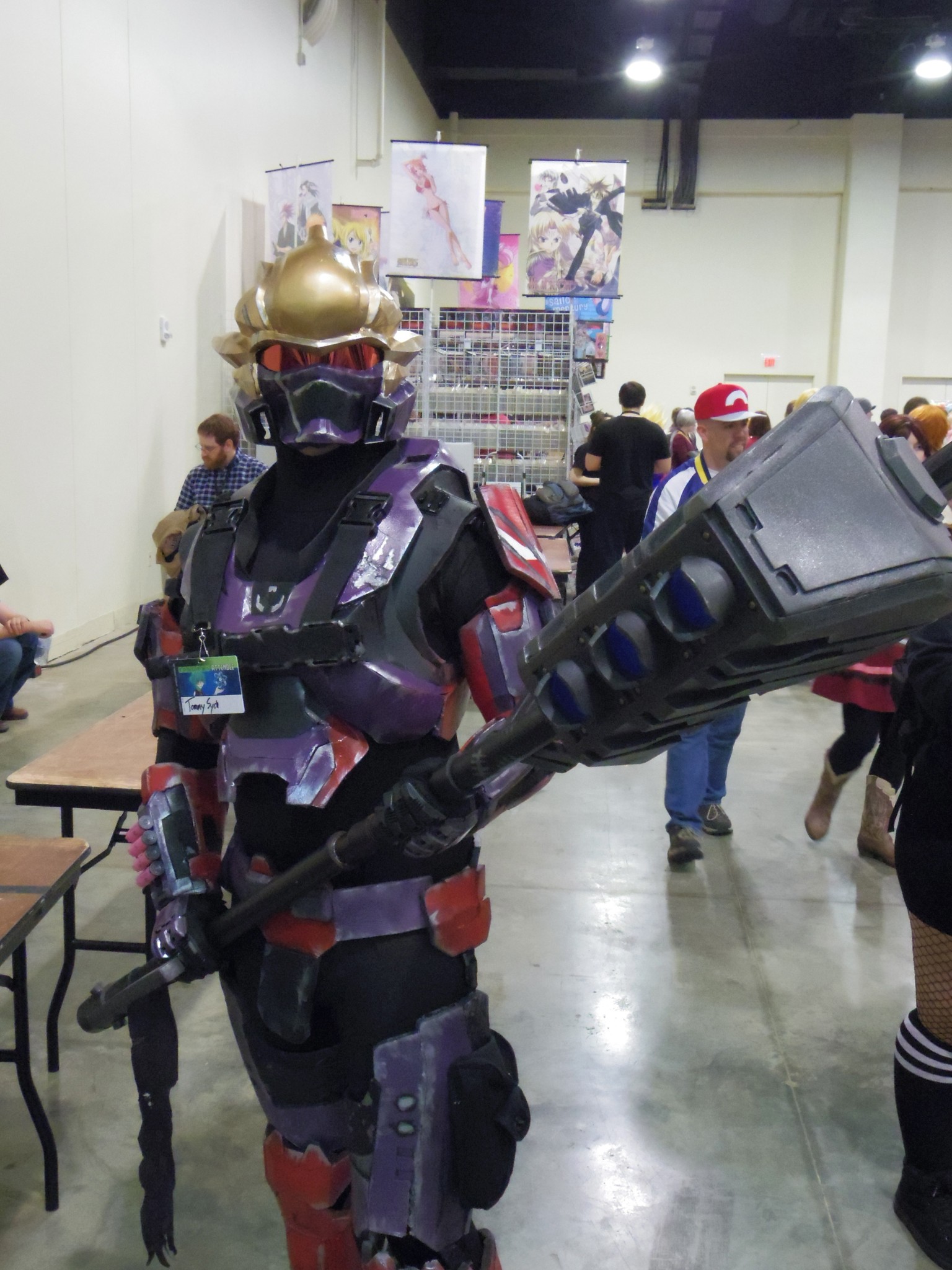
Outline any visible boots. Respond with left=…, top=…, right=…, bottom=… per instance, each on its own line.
left=894, top=1009, right=952, bottom=1270
left=858, top=775, right=895, bottom=868
left=804, top=750, right=854, bottom=840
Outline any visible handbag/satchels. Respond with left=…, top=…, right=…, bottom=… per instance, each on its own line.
left=524, top=481, right=593, bottom=527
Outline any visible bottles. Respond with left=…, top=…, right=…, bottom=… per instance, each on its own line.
left=34, top=633, right=52, bottom=666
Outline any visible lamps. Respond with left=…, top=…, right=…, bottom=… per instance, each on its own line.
left=916, top=19, right=952, bottom=81
left=624, top=35, right=664, bottom=83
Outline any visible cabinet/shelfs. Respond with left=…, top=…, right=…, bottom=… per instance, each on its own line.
left=396, top=310, right=581, bottom=500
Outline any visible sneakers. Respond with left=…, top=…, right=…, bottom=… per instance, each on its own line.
left=0, top=707, right=29, bottom=720
left=699, top=804, right=732, bottom=836
left=0, top=721, right=9, bottom=732
left=667, top=826, right=703, bottom=862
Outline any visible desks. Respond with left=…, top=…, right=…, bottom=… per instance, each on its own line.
left=6, top=684, right=161, bottom=1073
left=0, top=837, right=91, bottom=1212
left=530, top=522, right=579, bottom=608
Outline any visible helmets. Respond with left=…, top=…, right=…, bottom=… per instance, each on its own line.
left=213, top=216, right=424, bottom=448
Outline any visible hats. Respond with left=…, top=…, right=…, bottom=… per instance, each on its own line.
left=855, top=398, right=876, bottom=413
left=695, top=383, right=767, bottom=422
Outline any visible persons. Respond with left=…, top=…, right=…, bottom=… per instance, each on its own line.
left=173, top=414, right=268, bottom=511
left=889, top=618, right=952, bottom=1270
left=569, top=382, right=951, bottom=867
left=121, top=225, right=561, bottom=1270
left=0, top=564, right=54, bottom=732
